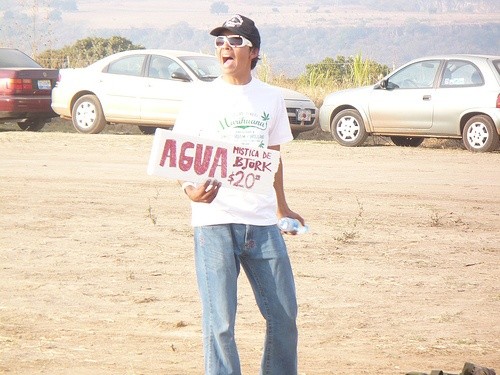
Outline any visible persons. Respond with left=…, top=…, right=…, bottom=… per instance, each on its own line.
left=170, top=14, right=307, bottom=375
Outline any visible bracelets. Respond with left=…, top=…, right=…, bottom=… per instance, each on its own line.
left=180, top=181, right=195, bottom=192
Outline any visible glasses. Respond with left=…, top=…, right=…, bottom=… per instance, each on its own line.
left=214, top=35, right=252, bottom=49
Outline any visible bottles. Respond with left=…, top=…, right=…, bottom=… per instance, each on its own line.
left=278, top=217, right=310, bottom=235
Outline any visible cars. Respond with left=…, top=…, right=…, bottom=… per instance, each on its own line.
left=0, top=48, right=58, bottom=133
left=50, top=49, right=319, bottom=144
left=319, top=54, right=500, bottom=153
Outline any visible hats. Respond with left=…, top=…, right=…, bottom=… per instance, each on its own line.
left=209, top=14, right=261, bottom=51
